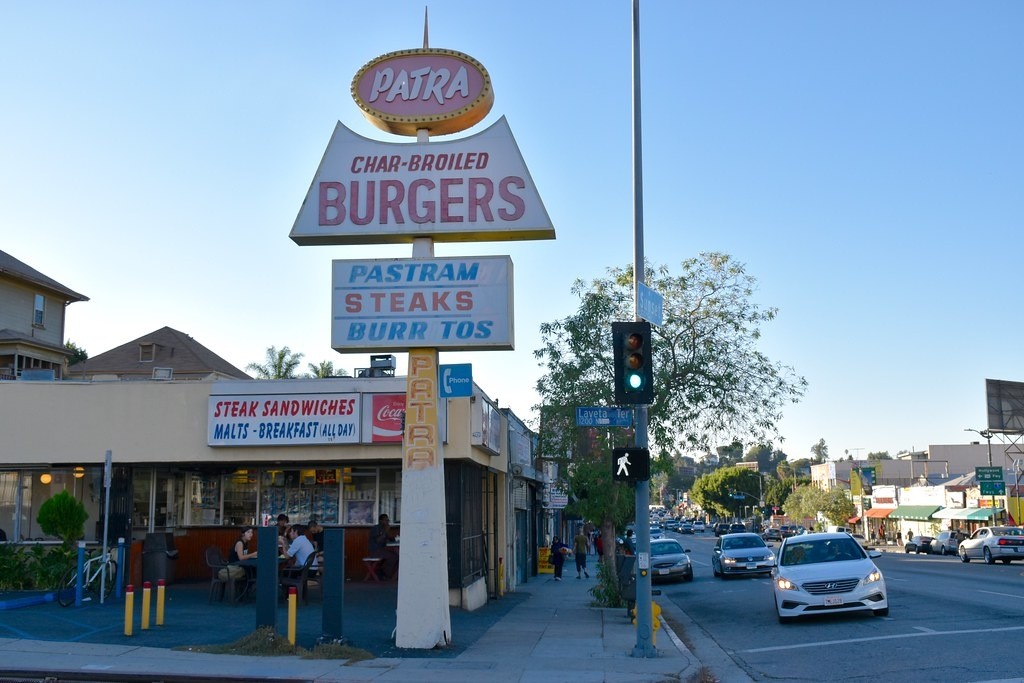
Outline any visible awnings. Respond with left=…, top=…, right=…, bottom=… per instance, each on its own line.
left=848, top=517, right=861, bottom=524
left=863, top=508, right=893, bottom=519
left=888, top=506, right=942, bottom=521
left=932, top=506, right=1005, bottom=520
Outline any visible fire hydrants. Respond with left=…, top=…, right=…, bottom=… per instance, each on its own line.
left=632, top=599, right=661, bottom=646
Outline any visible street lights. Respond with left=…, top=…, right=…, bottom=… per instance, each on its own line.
left=964, top=428, right=997, bottom=527
left=747, top=473, right=763, bottom=522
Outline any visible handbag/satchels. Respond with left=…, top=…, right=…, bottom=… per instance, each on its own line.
left=548, top=552, right=555, bottom=564
left=218, top=565, right=245, bottom=579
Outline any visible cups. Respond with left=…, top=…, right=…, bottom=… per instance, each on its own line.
left=262, top=513, right=269, bottom=527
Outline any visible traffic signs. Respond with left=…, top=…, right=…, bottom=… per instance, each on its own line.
left=975, top=466, right=1003, bottom=481
left=980, top=481, right=1006, bottom=495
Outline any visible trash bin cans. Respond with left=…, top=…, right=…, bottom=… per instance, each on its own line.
left=142, top=532, right=176, bottom=587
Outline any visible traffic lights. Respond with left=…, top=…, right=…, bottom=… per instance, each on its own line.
left=680, top=496, right=683, bottom=502
left=614, top=321, right=655, bottom=405
left=611, top=446, right=650, bottom=481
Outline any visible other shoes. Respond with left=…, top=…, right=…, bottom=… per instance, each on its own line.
left=584, top=572, right=589, bottom=578
left=577, top=576, right=581, bottom=579
left=556, top=577, right=562, bottom=581
left=376, top=572, right=390, bottom=581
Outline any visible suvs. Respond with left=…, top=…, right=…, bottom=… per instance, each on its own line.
left=930, top=531, right=969, bottom=555
left=958, top=527, right=1024, bottom=563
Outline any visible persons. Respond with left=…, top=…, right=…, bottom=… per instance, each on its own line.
left=808, top=527, right=816, bottom=534
left=572, top=528, right=590, bottom=579
left=551, top=536, right=568, bottom=581
left=826, top=542, right=851, bottom=561
left=367, top=514, right=399, bottom=581
left=896, top=530, right=902, bottom=546
left=792, top=547, right=804, bottom=561
left=744, top=541, right=753, bottom=547
left=587, top=529, right=605, bottom=563
left=879, top=522, right=886, bottom=541
left=624, top=529, right=634, bottom=555
left=906, top=528, right=913, bottom=541
left=230, top=526, right=257, bottom=600
left=274, top=513, right=325, bottom=600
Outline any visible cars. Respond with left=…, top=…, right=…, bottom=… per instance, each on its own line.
left=762, top=525, right=869, bottom=549
left=649, top=507, right=706, bottom=540
left=712, top=533, right=777, bottom=579
left=649, top=539, right=694, bottom=586
left=706, top=521, right=745, bottom=536
left=904, top=536, right=933, bottom=555
left=617, top=521, right=637, bottom=549
left=774, top=532, right=889, bottom=625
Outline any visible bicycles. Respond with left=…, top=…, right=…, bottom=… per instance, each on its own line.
left=57, top=539, right=131, bottom=607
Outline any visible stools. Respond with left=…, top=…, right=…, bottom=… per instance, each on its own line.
left=362, top=557, right=382, bottom=585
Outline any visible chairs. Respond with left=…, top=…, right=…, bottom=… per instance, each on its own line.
left=205, top=545, right=324, bottom=606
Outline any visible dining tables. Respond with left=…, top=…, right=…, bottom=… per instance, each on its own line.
left=387, top=542, right=400, bottom=582
left=231, top=556, right=287, bottom=605
